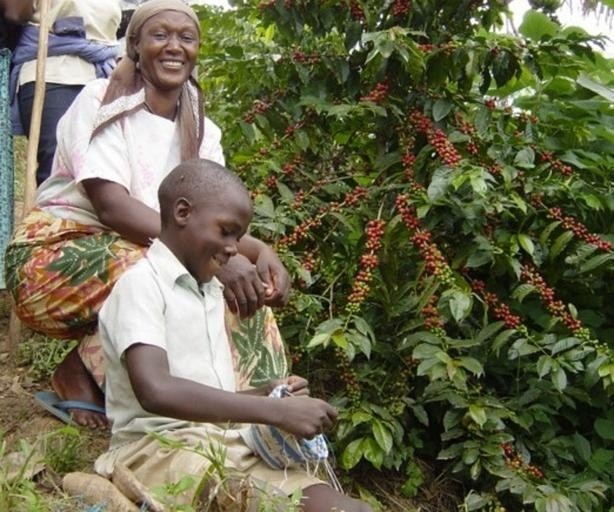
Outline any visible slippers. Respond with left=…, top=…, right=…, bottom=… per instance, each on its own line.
left=35, top=392, right=105, bottom=432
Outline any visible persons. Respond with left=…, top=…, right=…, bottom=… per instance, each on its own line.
left=8, top=0, right=293, bottom=431
left=97, top=158, right=378, bottom=512
left=1, top=0, right=127, bottom=192
left=0, top=0, right=17, bottom=351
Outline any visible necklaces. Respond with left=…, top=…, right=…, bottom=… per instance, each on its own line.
left=143, top=96, right=180, bottom=122
